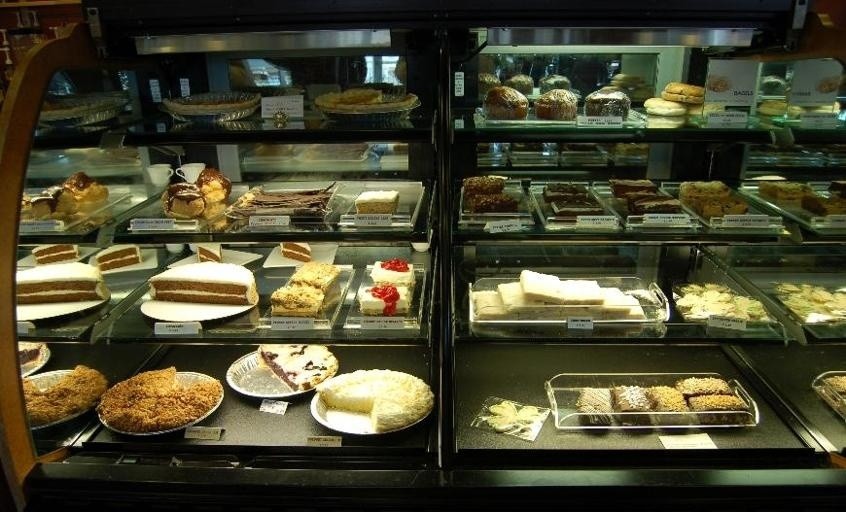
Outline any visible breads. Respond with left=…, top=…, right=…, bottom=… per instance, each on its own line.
left=40, top=100, right=108, bottom=117
left=478, top=72, right=651, bottom=102
left=461, top=177, right=746, bottom=219
left=757, top=99, right=840, bottom=116
left=163, top=93, right=261, bottom=116
left=776, top=282, right=846, bottom=319
left=759, top=181, right=846, bottom=215
left=484, top=82, right=727, bottom=128
left=825, top=375, right=846, bottom=395
left=473, top=270, right=767, bottom=323
left=579, top=377, right=749, bottom=426
left=315, top=87, right=419, bottom=115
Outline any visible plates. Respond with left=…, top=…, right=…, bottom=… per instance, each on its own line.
left=314, top=91, right=421, bottom=121
left=140, top=296, right=256, bottom=319
left=163, top=94, right=263, bottom=121
left=39, top=97, right=133, bottom=121
left=16, top=288, right=110, bottom=321
left=261, top=264, right=354, bottom=329
left=18, top=341, right=50, bottom=377
left=344, top=263, right=426, bottom=329
left=19, top=368, right=101, bottom=430
left=225, top=349, right=341, bottom=400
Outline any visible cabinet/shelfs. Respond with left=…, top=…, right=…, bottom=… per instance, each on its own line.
left=443, top=11, right=843, bottom=512
left=0, top=18, right=447, bottom=512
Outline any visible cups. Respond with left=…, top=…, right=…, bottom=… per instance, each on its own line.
left=175, top=162, right=206, bottom=184
left=146, top=164, right=174, bottom=187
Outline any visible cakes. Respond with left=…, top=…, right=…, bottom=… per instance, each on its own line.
left=257, top=344, right=338, bottom=393
left=476, top=142, right=650, bottom=155
left=149, top=167, right=415, bottom=316
left=13, top=171, right=141, bottom=305
left=16, top=341, right=43, bottom=375
left=20, top=365, right=108, bottom=428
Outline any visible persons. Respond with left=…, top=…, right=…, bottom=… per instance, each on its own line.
left=186, top=56, right=366, bottom=94
